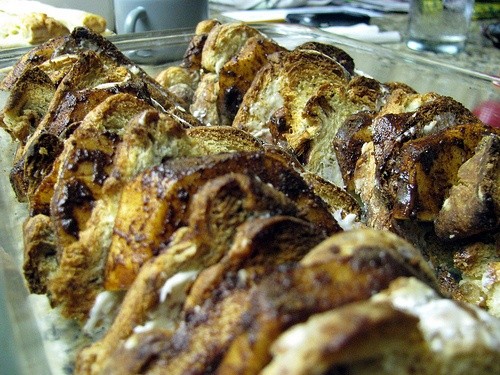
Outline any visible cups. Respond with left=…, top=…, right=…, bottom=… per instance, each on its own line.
left=406, top=0, right=474, bottom=56
left=111, top=0, right=208, bottom=63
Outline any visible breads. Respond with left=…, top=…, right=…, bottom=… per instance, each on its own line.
left=0, top=11, right=500, bottom=375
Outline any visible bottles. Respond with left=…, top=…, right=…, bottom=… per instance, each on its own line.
left=474, top=80, right=499, bottom=133
